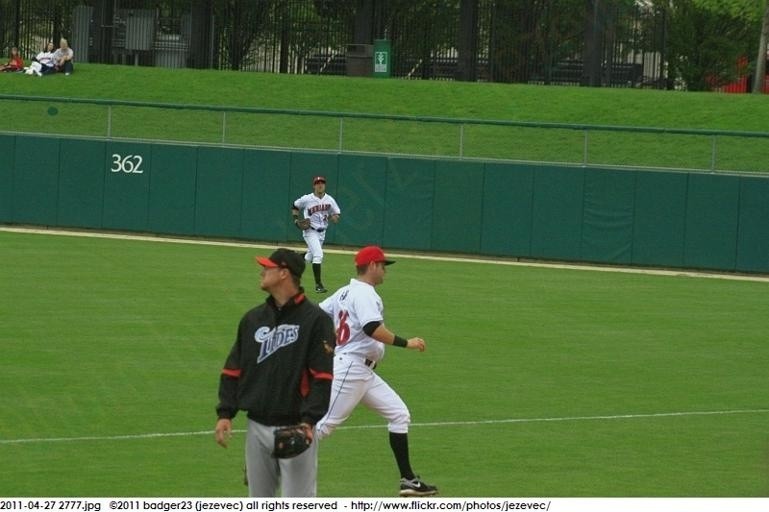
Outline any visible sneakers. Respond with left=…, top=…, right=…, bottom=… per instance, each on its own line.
left=314, top=281, right=328, bottom=293
left=398, top=475, right=438, bottom=496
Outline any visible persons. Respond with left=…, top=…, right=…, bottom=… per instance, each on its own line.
left=44, top=39, right=74, bottom=76
left=214, top=248, right=335, bottom=497
left=23, top=42, right=55, bottom=73
left=244, top=246, right=437, bottom=496
left=0, top=47, right=24, bottom=72
left=292, top=177, right=341, bottom=293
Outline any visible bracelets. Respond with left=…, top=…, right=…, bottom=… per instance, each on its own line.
left=392, top=335, right=407, bottom=347
left=293, top=215, right=298, bottom=221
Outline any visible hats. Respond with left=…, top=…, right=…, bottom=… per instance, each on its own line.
left=313, top=175, right=327, bottom=184
left=255, top=247, right=307, bottom=277
left=353, top=244, right=396, bottom=266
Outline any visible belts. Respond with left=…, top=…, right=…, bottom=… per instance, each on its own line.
left=362, top=357, right=377, bottom=370
left=309, top=225, right=327, bottom=233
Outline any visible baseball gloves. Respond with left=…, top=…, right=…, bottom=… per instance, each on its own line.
left=269, top=426, right=314, bottom=459
left=295, top=218, right=311, bottom=231
